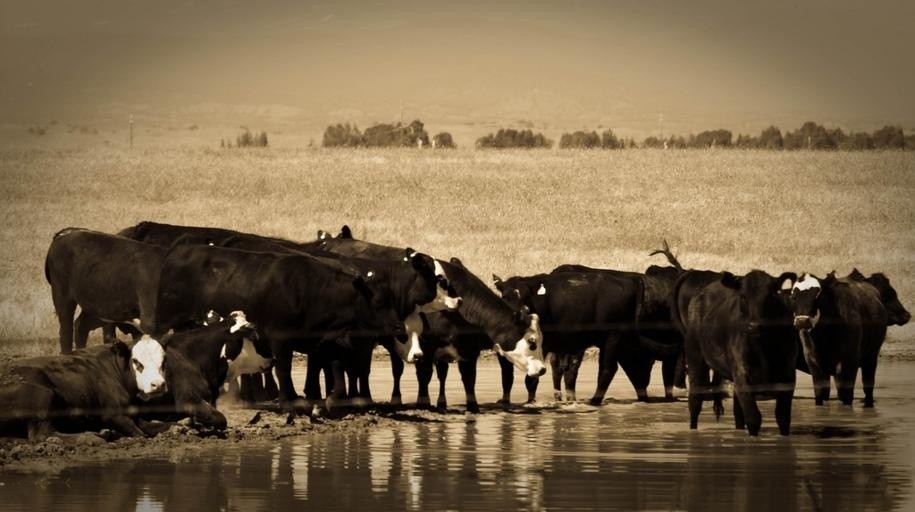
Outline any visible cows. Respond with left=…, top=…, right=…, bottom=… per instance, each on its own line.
left=0, top=221, right=912, bottom=444
